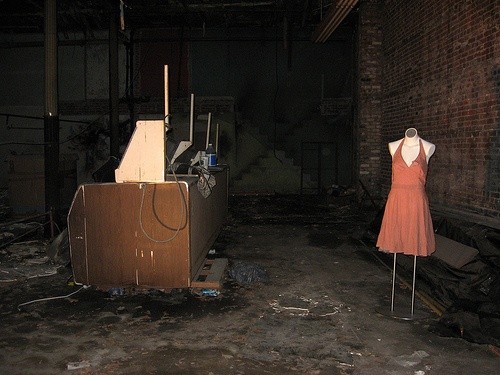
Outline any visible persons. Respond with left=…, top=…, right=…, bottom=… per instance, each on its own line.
left=123, top=120, right=135, bottom=146
left=376, top=128, right=436, bottom=256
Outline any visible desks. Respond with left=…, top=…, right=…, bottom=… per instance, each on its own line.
left=67, top=165, right=230, bottom=288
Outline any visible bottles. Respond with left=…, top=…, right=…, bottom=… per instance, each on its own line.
left=205, top=144, right=217, bottom=167
